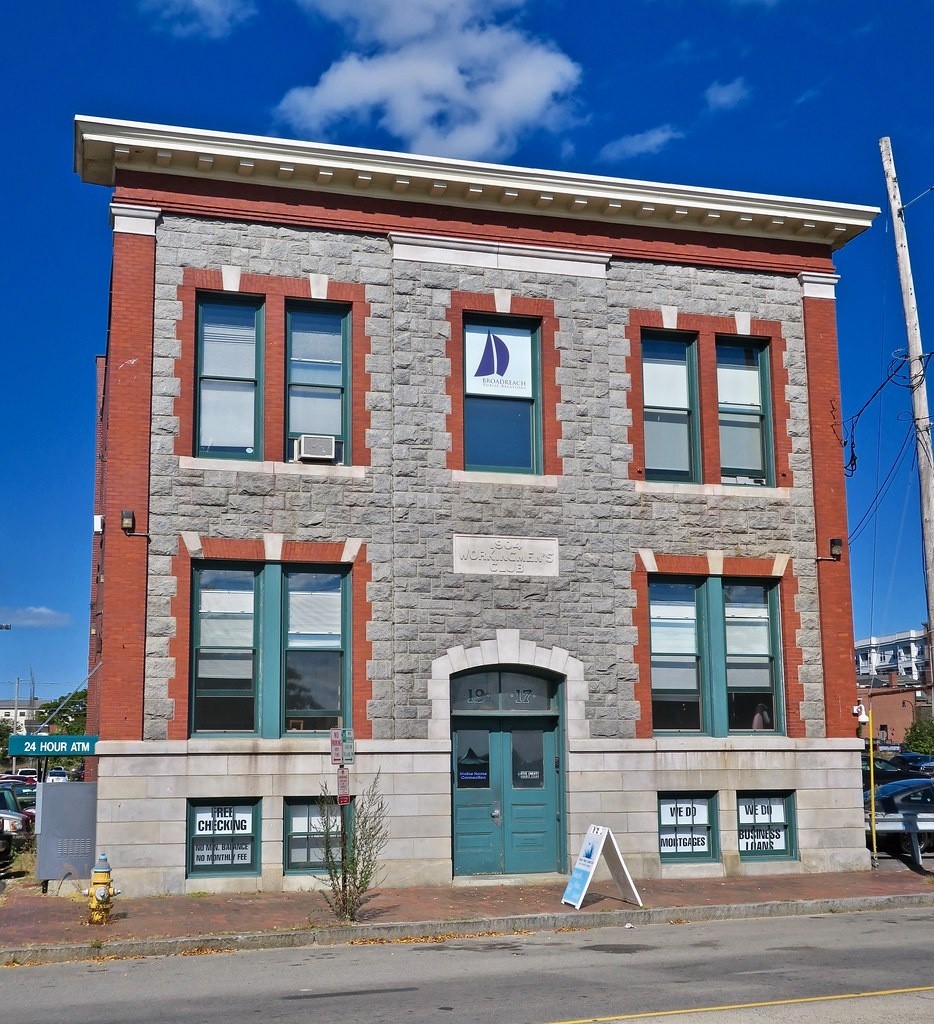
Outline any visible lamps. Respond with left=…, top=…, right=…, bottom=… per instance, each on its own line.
left=853, top=704, right=870, bottom=725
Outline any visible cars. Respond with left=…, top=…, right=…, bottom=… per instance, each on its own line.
left=47, top=770, right=68, bottom=783
left=861, top=755, right=933, bottom=794
left=887, top=753, right=934, bottom=779
left=16, top=768, right=38, bottom=782
left=51, top=765, right=65, bottom=771
left=71, top=763, right=85, bottom=782
left=863, top=778, right=934, bottom=855
left=0, top=773, right=37, bottom=871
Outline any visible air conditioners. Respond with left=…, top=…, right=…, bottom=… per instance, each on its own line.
left=296, top=434, right=336, bottom=461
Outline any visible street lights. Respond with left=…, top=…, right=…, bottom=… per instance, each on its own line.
left=902, top=699, right=915, bottom=725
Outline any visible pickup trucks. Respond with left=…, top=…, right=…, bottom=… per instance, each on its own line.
left=863, top=737, right=902, bottom=754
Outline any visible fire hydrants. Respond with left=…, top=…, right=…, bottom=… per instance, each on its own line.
left=81, top=853, right=121, bottom=927
